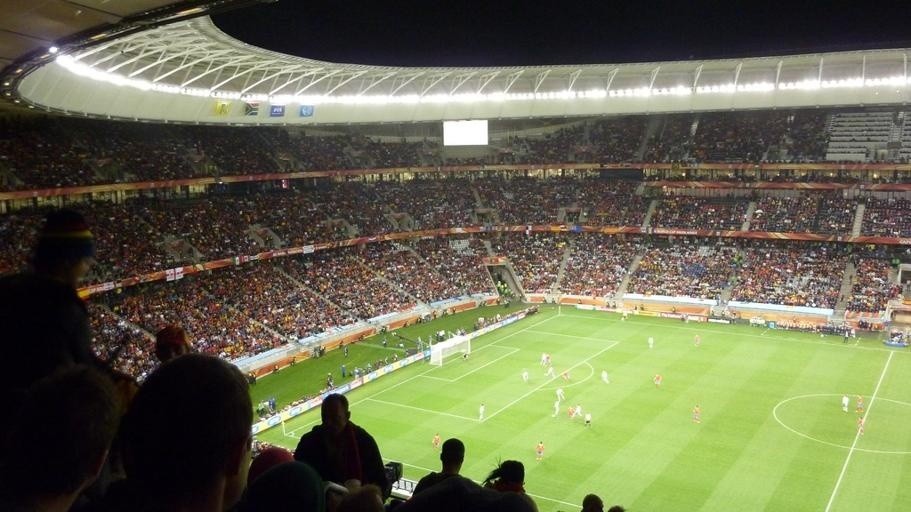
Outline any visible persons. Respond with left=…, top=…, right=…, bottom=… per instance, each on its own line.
left=5, top=106, right=911, bottom=512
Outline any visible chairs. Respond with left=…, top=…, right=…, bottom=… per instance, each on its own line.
left=661, top=112, right=911, bottom=315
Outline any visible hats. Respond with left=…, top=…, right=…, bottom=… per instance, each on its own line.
left=246, top=448, right=295, bottom=488
